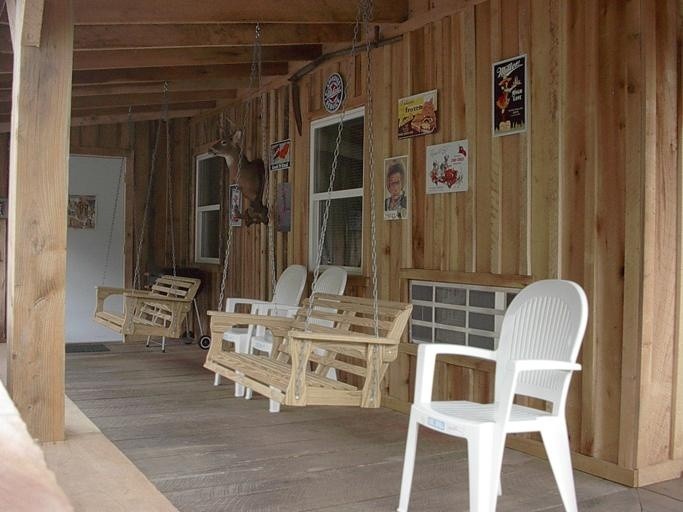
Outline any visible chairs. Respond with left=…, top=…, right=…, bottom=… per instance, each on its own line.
left=244, top=267, right=347, bottom=413
left=397, top=279, right=589, bottom=512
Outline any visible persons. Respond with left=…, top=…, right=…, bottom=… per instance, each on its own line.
left=385, top=163, right=407, bottom=211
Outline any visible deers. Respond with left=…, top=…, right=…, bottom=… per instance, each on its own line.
left=205, top=111, right=265, bottom=210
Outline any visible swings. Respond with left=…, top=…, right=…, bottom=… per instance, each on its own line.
left=202, top=5, right=411, bottom=406
left=91, top=81, right=201, bottom=339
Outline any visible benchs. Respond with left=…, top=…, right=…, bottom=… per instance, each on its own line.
left=202, top=293, right=413, bottom=408
left=92, top=274, right=202, bottom=338
left=214, top=264, right=308, bottom=397
